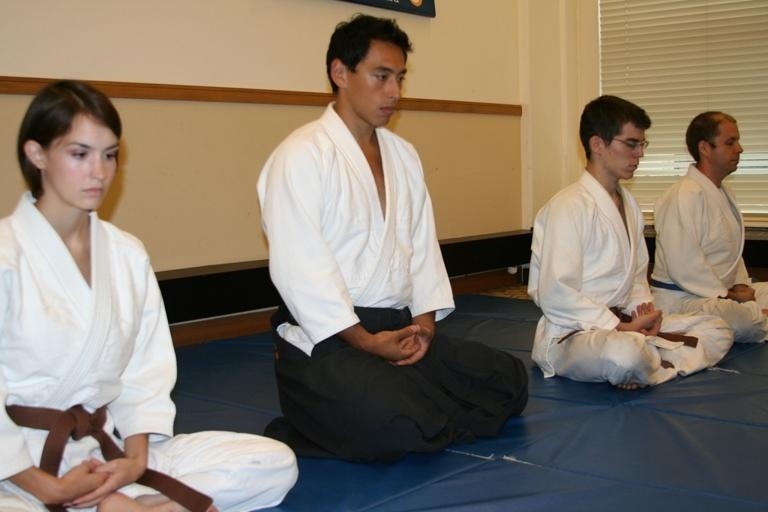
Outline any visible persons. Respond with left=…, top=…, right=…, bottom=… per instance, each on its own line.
left=0, top=77, right=301, bottom=512
left=647, top=110, right=768, bottom=345
left=524, top=94, right=733, bottom=391
left=251, top=10, right=529, bottom=465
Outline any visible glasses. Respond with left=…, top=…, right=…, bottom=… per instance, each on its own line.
left=602, top=133, right=650, bottom=150
